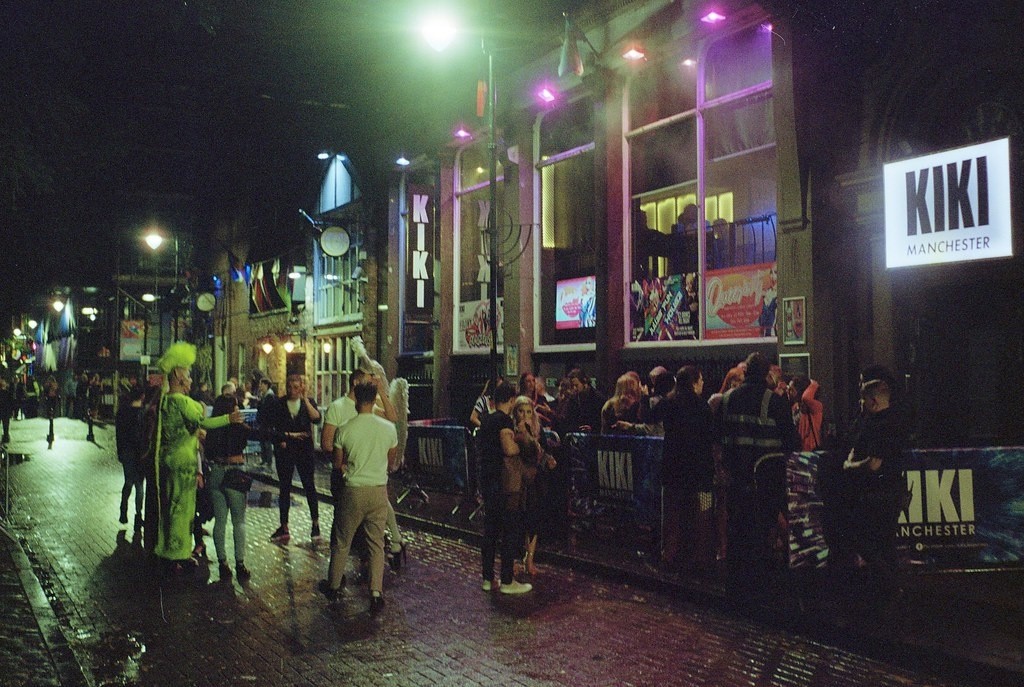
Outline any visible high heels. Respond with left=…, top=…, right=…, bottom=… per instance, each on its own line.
left=192, top=544, right=206, bottom=557
left=387, top=542, right=408, bottom=569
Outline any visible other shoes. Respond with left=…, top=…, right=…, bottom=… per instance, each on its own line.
left=271, top=526, right=291, bottom=541
left=481, top=580, right=491, bottom=592
left=318, top=579, right=340, bottom=600
left=310, top=524, right=321, bottom=539
left=370, top=596, right=385, bottom=610
left=523, top=566, right=545, bottom=576
left=218, top=565, right=232, bottom=579
left=234, top=561, right=250, bottom=578
left=499, top=579, right=533, bottom=594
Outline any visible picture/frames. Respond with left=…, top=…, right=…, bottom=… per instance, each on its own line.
left=779, top=352, right=811, bottom=394
left=782, top=296, right=807, bottom=346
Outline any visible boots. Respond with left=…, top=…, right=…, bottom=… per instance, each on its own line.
left=119, top=504, right=128, bottom=524
left=134, top=514, right=142, bottom=531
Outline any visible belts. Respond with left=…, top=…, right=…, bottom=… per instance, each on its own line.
left=208, top=459, right=244, bottom=466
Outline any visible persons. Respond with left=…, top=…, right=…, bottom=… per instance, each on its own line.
left=116, top=336, right=410, bottom=609
left=470, top=351, right=907, bottom=595
left=0, top=373, right=103, bottom=443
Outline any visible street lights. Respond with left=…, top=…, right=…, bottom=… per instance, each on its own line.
left=420, top=7, right=500, bottom=401
left=136, top=225, right=182, bottom=343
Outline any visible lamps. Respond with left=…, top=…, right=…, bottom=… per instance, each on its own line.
left=276, top=328, right=307, bottom=354
left=255, top=330, right=275, bottom=355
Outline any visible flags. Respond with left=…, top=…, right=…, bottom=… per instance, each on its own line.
left=228, top=250, right=251, bottom=288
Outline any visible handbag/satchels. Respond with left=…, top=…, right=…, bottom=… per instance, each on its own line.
left=222, top=469, right=253, bottom=492
